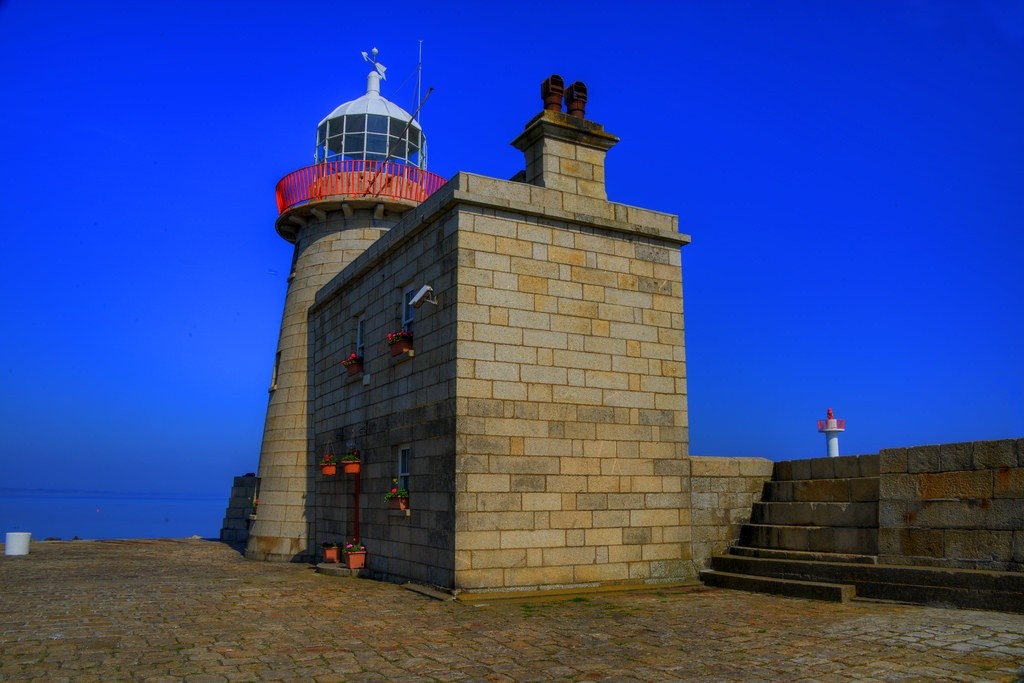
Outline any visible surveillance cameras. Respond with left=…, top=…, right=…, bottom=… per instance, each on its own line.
left=408, top=285, right=433, bottom=309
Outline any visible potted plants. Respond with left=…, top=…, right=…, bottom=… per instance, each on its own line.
left=319, top=542, right=341, bottom=563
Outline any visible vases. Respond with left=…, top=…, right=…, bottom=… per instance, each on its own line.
left=342, top=461, right=361, bottom=474
left=387, top=497, right=407, bottom=510
left=346, top=364, right=360, bottom=376
left=320, top=463, right=336, bottom=475
left=345, top=551, right=366, bottom=570
left=389, top=340, right=410, bottom=356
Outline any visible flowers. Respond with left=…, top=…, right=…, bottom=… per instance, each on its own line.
left=341, top=353, right=363, bottom=365
left=341, top=452, right=361, bottom=461
left=343, top=538, right=364, bottom=552
left=319, top=454, right=338, bottom=464
left=383, top=478, right=409, bottom=503
left=387, top=330, right=418, bottom=346
